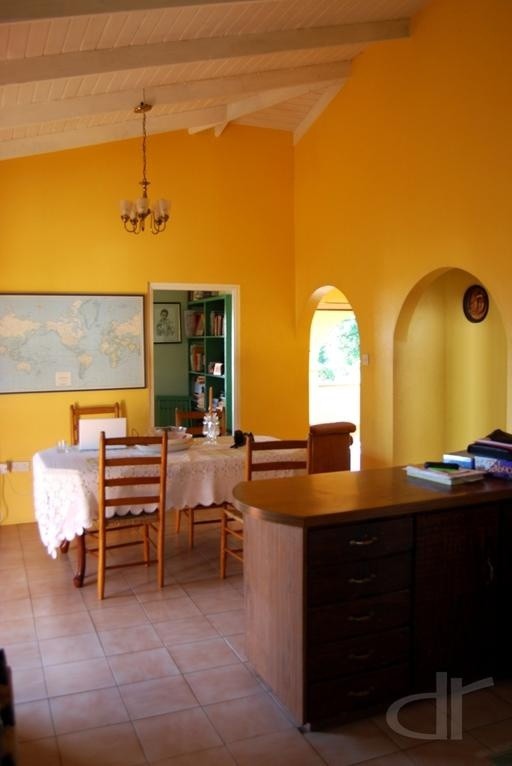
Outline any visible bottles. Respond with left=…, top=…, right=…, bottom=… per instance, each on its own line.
left=234, top=429, right=247, bottom=446
left=197, top=353, right=204, bottom=372
left=57, top=439, right=67, bottom=453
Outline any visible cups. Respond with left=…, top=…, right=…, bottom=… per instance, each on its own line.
left=183, top=310, right=196, bottom=336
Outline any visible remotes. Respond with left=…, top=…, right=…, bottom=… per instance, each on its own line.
left=424, top=461, right=458, bottom=470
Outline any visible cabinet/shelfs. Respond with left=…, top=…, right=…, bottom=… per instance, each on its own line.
left=184, top=293, right=232, bottom=432
left=231, top=461, right=512, bottom=734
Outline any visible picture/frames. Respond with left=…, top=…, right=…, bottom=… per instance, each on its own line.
left=0, top=292, right=147, bottom=396
left=153, top=301, right=182, bottom=345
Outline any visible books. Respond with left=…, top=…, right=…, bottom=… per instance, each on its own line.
left=403, top=428, right=511, bottom=487
left=186, top=305, right=225, bottom=410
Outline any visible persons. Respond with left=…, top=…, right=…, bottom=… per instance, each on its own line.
left=155, top=310, right=174, bottom=337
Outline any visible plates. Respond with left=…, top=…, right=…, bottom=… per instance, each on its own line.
left=135, top=426, right=193, bottom=451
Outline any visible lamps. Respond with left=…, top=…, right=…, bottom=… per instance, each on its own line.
left=118, top=84, right=170, bottom=236
